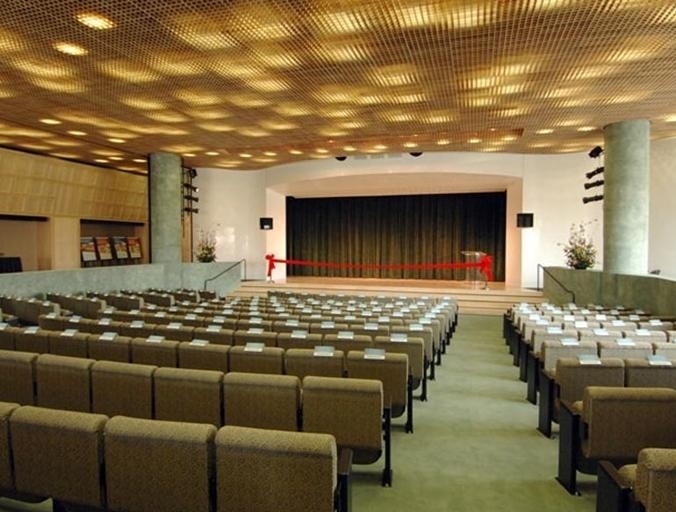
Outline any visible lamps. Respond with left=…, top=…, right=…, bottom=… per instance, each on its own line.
left=585, top=166, right=604, bottom=179
left=183, top=182, right=199, bottom=193
left=184, top=207, right=199, bottom=214
left=588, top=146, right=603, bottom=158
left=187, top=168, right=198, bottom=180
left=584, top=180, right=604, bottom=190
left=184, top=195, right=200, bottom=203
left=583, top=195, right=603, bottom=204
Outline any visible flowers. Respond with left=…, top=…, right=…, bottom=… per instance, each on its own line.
left=193, top=229, right=217, bottom=264
left=556, top=223, right=597, bottom=269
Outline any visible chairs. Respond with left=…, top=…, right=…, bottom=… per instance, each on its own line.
left=500, top=296, right=676, bottom=404
left=59, top=317, right=92, bottom=333
left=156, top=288, right=460, bottom=355
left=535, top=357, right=626, bottom=439
left=0, top=349, right=39, bottom=409
left=162, top=307, right=186, bottom=316
left=7, top=405, right=109, bottom=512
left=140, top=307, right=163, bottom=314
left=101, top=416, right=218, bottom=512
left=553, top=387, right=675, bottom=497
left=0, top=401, right=21, bottom=512
left=215, top=425, right=352, bottom=512
left=594, top=448, right=676, bottom=512
left=300, top=375, right=393, bottom=488
left=0, top=309, right=17, bottom=323
left=119, top=322, right=156, bottom=339
left=284, top=349, right=344, bottom=394
left=96, top=309, right=121, bottom=322
left=13, top=328, right=52, bottom=356
left=86, top=335, right=132, bottom=364
left=152, top=367, right=224, bottom=431
left=86, top=319, right=122, bottom=337
left=0, top=326, right=19, bottom=351
left=221, top=372, right=299, bottom=432
left=390, top=326, right=435, bottom=382
left=38, top=314, right=64, bottom=331
left=374, top=336, right=428, bottom=402
left=345, top=350, right=413, bottom=435
left=228, top=345, right=285, bottom=375
left=130, top=337, right=180, bottom=369
left=35, top=353, right=97, bottom=416
left=177, top=342, right=232, bottom=375
left=174, top=300, right=195, bottom=309
left=403, top=320, right=441, bottom=366
left=145, top=313, right=174, bottom=324
left=120, top=312, right=145, bottom=324
left=89, top=360, right=158, bottom=420
left=47, top=331, right=91, bottom=359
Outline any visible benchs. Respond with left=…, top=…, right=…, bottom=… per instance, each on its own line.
left=44, top=292, right=115, bottom=318
left=0, top=294, right=73, bottom=324
left=84, top=290, right=157, bottom=311
left=119, top=288, right=176, bottom=307
left=148, top=288, right=200, bottom=302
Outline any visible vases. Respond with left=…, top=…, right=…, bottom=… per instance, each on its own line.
left=573, top=266, right=586, bottom=270
left=198, top=259, right=212, bottom=263
